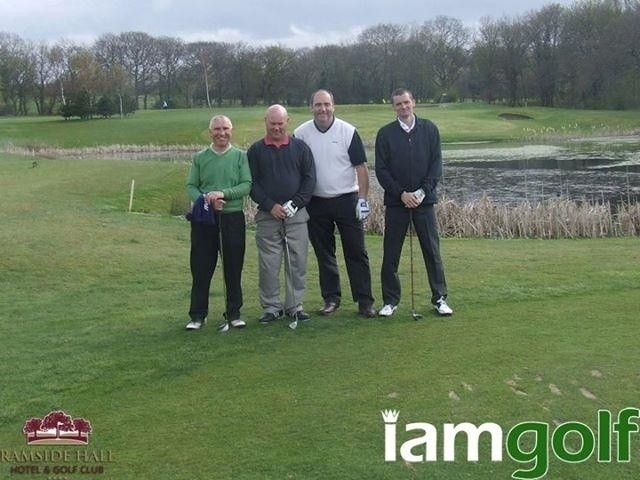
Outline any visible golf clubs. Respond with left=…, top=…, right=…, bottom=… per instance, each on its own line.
left=409, top=208, right=422, bottom=319
left=281, top=218, right=298, bottom=329
left=217, top=211, right=229, bottom=331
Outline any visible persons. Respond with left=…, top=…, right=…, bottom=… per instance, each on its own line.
left=293, top=88, right=379, bottom=318
left=375, top=87, right=455, bottom=317
left=183, top=114, right=253, bottom=332
left=246, top=103, right=316, bottom=325
left=160, top=99, right=169, bottom=110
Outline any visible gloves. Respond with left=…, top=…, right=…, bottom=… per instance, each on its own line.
left=282, top=196, right=304, bottom=218
left=356, top=199, right=371, bottom=222
left=405, top=189, right=426, bottom=208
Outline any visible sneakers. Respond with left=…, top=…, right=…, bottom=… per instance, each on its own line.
left=259, top=309, right=284, bottom=324
left=230, top=319, right=245, bottom=329
left=433, top=297, right=453, bottom=315
left=379, top=305, right=397, bottom=317
left=285, top=310, right=311, bottom=322
left=186, top=319, right=206, bottom=330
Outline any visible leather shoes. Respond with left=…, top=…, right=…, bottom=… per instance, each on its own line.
left=319, top=302, right=337, bottom=316
left=359, top=308, right=377, bottom=317
